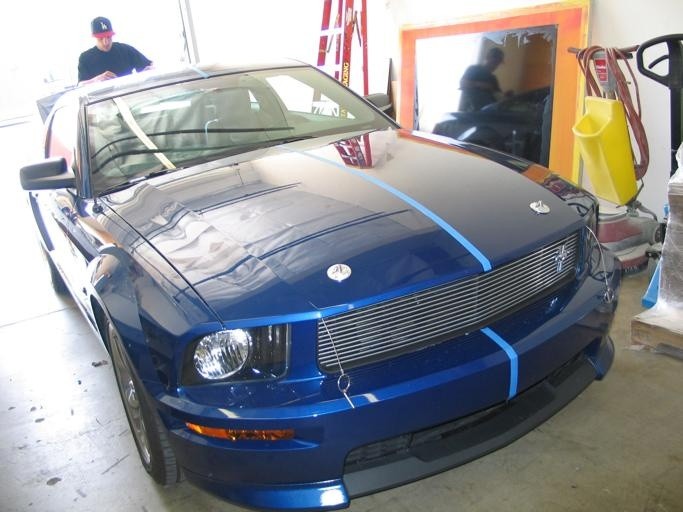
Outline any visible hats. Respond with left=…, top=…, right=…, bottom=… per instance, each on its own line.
left=426, top=85, right=562, bottom=178
left=16, top=49, right=628, bottom=512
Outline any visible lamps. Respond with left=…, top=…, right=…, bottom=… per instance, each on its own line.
left=204, top=88, right=268, bottom=147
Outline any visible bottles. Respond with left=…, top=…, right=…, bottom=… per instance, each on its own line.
left=90, top=17, right=115, bottom=38
left=489, top=48, right=506, bottom=64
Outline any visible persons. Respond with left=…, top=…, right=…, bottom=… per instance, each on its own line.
left=76, top=15, right=154, bottom=89
left=456, top=45, right=516, bottom=113
